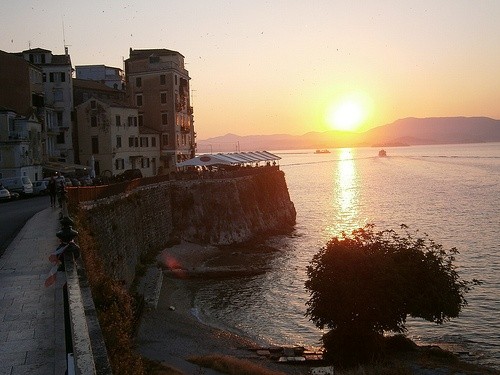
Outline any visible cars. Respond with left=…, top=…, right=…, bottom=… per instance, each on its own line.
left=0, top=186, right=21, bottom=203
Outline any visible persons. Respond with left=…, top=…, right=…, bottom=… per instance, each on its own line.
left=48, top=171, right=66, bottom=208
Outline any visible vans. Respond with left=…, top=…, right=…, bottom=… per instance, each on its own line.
left=0, top=176, right=33, bottom=196
left=32, top=176, right=65, bottom=197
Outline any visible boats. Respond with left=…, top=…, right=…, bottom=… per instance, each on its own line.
left=378, top=150, right=387, bottom=157
left=316, top=149, right=332, bottom=154
